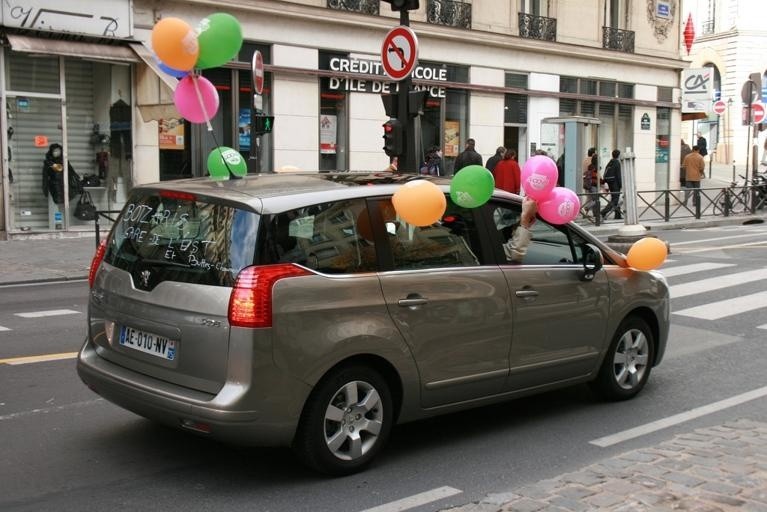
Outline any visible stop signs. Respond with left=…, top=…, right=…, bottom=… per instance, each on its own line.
left=252, top=50, right=264, bottom=95
left=713, top=101, right=727, bottom=115
left=751, top=104, right=766, bottom=124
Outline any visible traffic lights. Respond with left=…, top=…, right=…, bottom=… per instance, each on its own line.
left=381, top=91, right=431, bottom=157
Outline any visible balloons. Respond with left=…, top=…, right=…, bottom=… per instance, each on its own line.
left=207, top=146, right=247, bottom=180
left=521, top=155, right=558, bottom=201
left=148, top=39, right=188, bottom=78
left=391, top=179, right=447, bottom=227
left=173, top=73, right=220, bottom=123
left=449, top=164, right=496, bottom=208
left=195, top=13, right=243, bottom=69
left=151, top=17, right=199, bottom=72
left=626, top=237, right=667, bottom=271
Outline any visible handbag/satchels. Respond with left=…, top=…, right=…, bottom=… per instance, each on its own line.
left=73, top=190, right=99, bottom=220
left=80, top=174, right=101, bottom=187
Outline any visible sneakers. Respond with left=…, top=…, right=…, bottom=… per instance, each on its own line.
left=615, top=215, right=623, bottom=219
left=580, top=207, right=588, bottom=217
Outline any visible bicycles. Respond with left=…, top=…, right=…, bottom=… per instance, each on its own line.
left=713, top=174, right=754, bottom=216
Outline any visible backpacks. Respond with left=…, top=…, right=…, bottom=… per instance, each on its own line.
left=582, top=169, right=592, bottom=190
left=604, top=166, right=617, bottom=184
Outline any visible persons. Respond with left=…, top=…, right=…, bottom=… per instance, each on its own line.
left=502, top=196, right=541, bottom=264
left=454, top=138, right=483, bottom=176
left=420, top=145, right=443, bottom=177
left=531, top=130, right=709, bottom=226
left=491, top=148, right=521, bottom=195
left=383, top=156, right=398, bottom=171
left=485, top=146, right=507, bottom=179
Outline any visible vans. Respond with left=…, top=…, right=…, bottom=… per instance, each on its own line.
left=76, top=172, right=671, bottom=474
